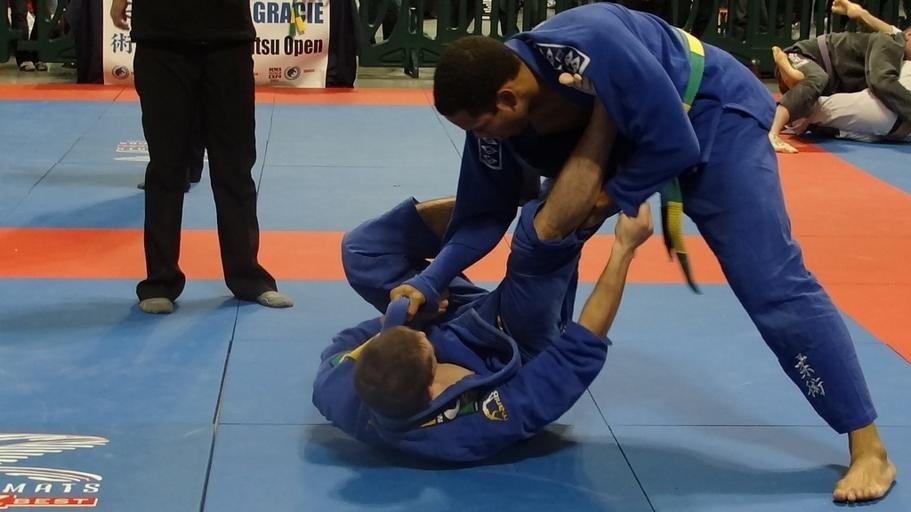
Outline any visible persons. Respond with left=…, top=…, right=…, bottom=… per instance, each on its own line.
left=110, top=0, right=298, bottom=316
left=389, top=0, right=900, bottom=504
left=8, top=0, right=51, bottom=73
left=773, top=1, right=911, bottom=140
left=766, top=25, right=911, bottom=154
left=312, top=97, right=654, bottom=472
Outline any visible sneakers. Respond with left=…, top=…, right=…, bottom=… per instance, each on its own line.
left=20, top=61, right=48, bottom=71
